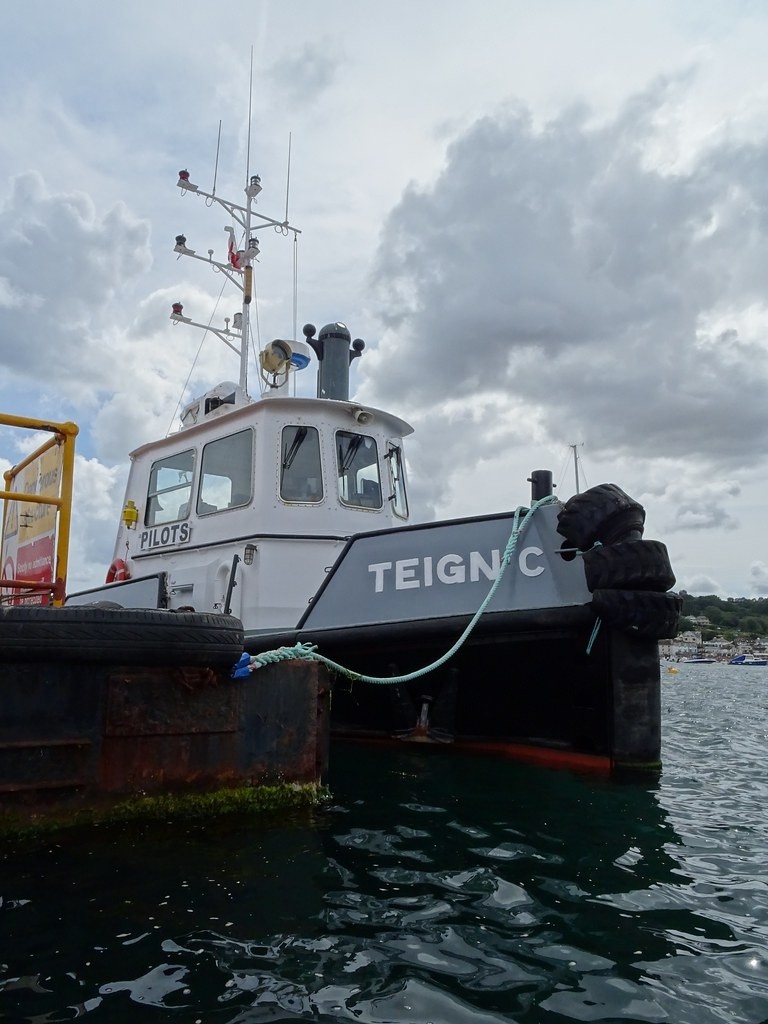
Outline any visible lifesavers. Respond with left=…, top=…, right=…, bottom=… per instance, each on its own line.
left=0, top=604, right=246, bottom=668
left=556, top=482, right=682, bottom=640
left=104, top=558, right=130, bottom=584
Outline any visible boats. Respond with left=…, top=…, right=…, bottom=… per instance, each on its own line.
left=668, top=669, right=678, bottom=674
left=684, top=653, right=717, bottom=665
left=728, top=652, right=767, bottom=665
left=58, top=171, right=681, bottom=800
left=719, top=640, right=768, bottom=654
